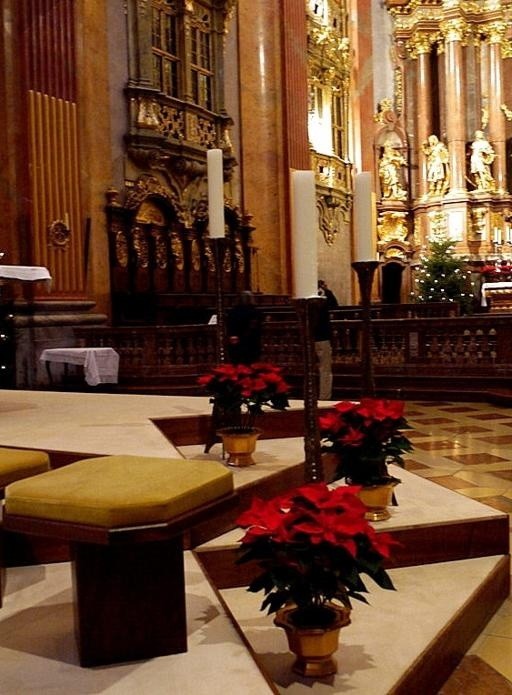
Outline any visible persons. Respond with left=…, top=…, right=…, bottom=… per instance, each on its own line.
left=224, top=291, right=261, bottom=364
left=378, top=141, right=407, bottom=197
left=308, top=298, right=334, bottom=400
left=425, top=135, right=448, bottom=194
left=469, top=130, right=495, bottom=190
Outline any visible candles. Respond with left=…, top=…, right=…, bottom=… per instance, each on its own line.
left=206, top=148, right=225, bottom=236
left=288, top=169, right=319, bottom=299
left=353, top=170, right=376, bottom=262
left=494, top=226, right=512, bottom=245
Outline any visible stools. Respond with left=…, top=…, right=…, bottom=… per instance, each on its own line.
left=0, top=445, right=54, bottom=567
left=3, top=455, right=238, bottom=668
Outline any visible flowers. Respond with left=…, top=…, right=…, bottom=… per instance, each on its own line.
left=307, top=395, right=415, bottom=506
left=195, top=362, right=293, bottom=418
left=480, top=264, right=512, bottom=283
left=234, top=478, right=405, bottom=616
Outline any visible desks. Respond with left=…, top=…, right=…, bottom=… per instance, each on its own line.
left=39, top=345, right=120, bottom=394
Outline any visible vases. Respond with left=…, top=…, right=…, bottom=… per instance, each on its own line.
left=273, top=602, right=351, bottom=678
left=215, top=427, right=264, bottom=466
left=344, top=476, right=398, bottom=522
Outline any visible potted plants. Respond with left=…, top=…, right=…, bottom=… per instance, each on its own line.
left=407, top=236, right=475, bottom=334
